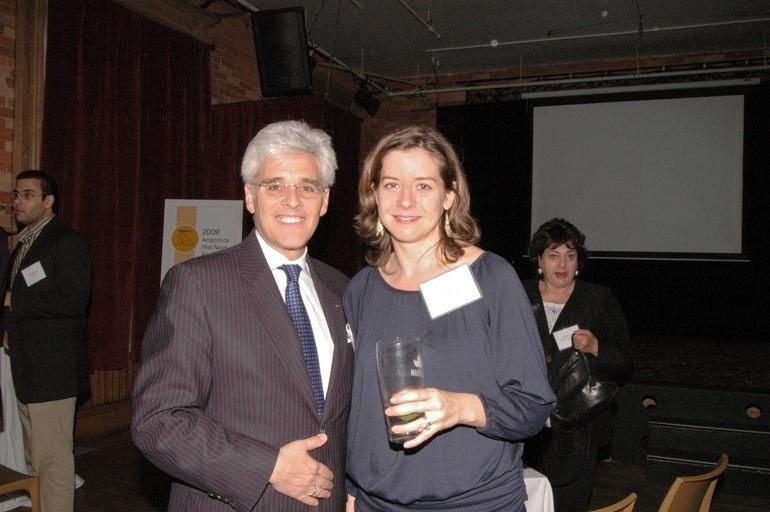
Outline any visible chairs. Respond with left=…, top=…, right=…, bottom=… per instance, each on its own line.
left=655, top=452, right=731, bottom=512
left=591, top=492, right=638, bottom=512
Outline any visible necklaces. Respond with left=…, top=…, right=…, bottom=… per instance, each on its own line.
left=551, top=296, right=564, bottom=314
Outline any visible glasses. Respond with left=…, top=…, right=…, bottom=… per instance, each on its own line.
left=13, top=190, right=40, bottom=201
left=252, top=180, right=323, bottom=202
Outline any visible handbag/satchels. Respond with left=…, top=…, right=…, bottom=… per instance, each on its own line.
left=550, top=345, right=621, bottom=435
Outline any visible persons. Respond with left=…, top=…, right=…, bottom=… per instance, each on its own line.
left=129, top=117, right=353, bottom=511
left=337, top=125, right=559, bottom=511
left=520, top=222, right=635, bottom=511
left=0, top=169, right=92, bottom=510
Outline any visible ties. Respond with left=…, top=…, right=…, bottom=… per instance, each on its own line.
left=278, top=265, right=323, bottom=416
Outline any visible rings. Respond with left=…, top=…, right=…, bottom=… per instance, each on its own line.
left=310, top=486, right=317, bottom=497
left=423, top=413, right=432, bottom=426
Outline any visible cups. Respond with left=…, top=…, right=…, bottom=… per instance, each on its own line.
left=375, top=334, right=423, bottom=444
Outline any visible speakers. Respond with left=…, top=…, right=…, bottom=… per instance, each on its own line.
left=251, top=6, right=314, bottom=98
